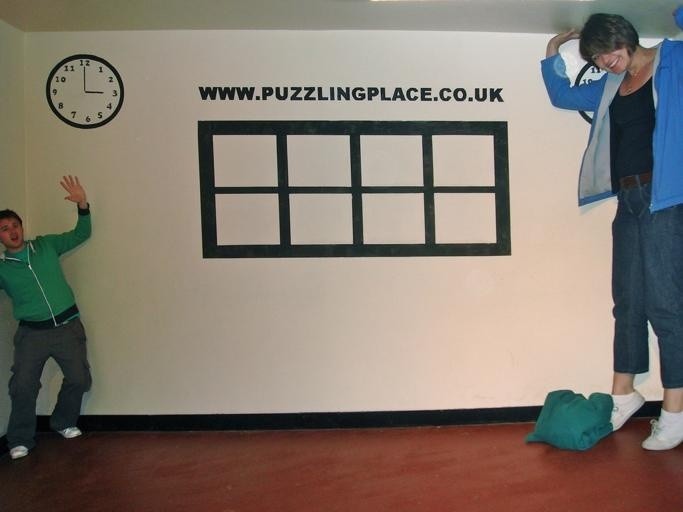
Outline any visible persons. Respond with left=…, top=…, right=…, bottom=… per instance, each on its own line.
left=0, top=173, right=92, bottom=462
left=539, top=3, right=682, bottom=451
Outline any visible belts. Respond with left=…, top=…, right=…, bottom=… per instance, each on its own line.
left=616, top=170, right=653, bottom=189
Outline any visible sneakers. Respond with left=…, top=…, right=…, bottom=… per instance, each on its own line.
left=7, top=444, right=26, bottom=462
left=54, top=426, right=82, bottom=439
left=604, top=388, right=646, bottom=431
left=636, top=407, right=682, bottom=451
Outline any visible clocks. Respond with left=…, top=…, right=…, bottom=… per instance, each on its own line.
left=46, top=54, right=125, bottom=129
left=574, top=64, right=607, bottom=122
